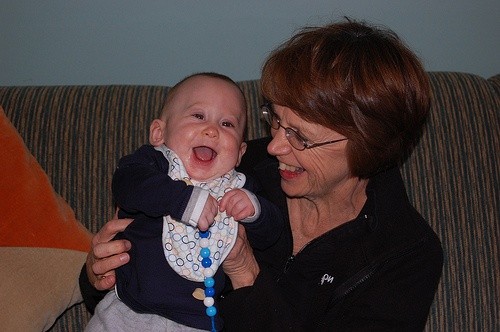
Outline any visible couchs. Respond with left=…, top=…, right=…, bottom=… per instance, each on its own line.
left=0, top=71, right=500, bottom=331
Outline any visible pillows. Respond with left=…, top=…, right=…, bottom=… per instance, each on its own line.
left=0, top=102, right=100, bottom=332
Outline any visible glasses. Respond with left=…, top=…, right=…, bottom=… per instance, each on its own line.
left=259, top=102, right=349, bottom=151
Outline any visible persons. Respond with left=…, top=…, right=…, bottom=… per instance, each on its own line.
left=78, top=22, right=441, bottom=332
left=88, top=72, right=262, bottom=332
